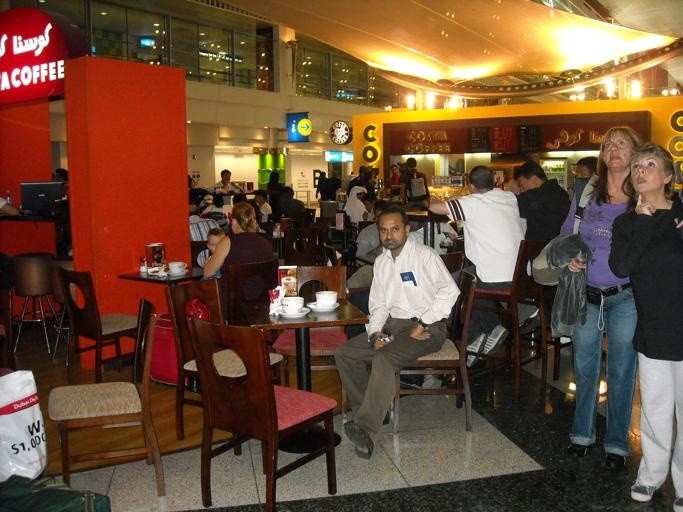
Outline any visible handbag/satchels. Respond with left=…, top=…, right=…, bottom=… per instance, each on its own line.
left=532, top=239, right=560, bottom=285
left=0, top=475, right=111, bottom=512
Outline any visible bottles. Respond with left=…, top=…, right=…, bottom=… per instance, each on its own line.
left=140, top=256, right=146, bottom=273
left=430, top=176, right=464, bottom=187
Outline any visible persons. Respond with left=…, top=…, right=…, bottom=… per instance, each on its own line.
left=1, top=169, right=71, bottom=321
left=188, top=169, right=330, bottom=322
left=329, top=122, right=683, bottom=512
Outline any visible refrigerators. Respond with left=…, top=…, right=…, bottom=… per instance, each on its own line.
left=538, top=157, right=575, bottom=201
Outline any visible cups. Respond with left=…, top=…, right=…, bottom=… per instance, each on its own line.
left=280, top=297, right=304, bottom=313
left=168, top=261, right=186, bottom=272
left=314, top=290, right=337, bottom=305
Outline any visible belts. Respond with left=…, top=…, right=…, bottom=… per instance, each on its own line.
left=607, top=284, right=632, bottom=297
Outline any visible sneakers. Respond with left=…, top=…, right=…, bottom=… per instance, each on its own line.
left=672, top=498, right=683, bottom=510
left=520, top=312, right=541, bottom=336
left=606, top=452, right=627, bottom=469
left=468, top=334, right=485, bottom=367
left=485, top=326, right=506, bottom=357
left=629, top=480, right=655, bottom=502
left=567, top=442, right=588, bottom=457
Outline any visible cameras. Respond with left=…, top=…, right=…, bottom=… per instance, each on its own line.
left=379, top=334, right=395, bottom=343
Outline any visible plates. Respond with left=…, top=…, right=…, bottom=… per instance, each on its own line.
left=276, top=308, right=311, bottom=319
left=308, top=302, right=340, bottom=312
left=167, top=270, right=190, bottom=277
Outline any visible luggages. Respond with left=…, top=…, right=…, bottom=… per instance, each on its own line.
left=148, top=314, right=188, bottom=386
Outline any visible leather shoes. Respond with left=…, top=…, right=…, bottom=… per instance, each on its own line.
left=344, top=421, right=374, bottom=458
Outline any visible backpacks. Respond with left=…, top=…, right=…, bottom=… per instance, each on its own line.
left=407, top=171, right=426, bottom=197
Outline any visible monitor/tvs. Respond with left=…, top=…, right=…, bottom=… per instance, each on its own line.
left=138, top=36, right=156, bottom=49
left=21, top=182, right=62, bottom=212
